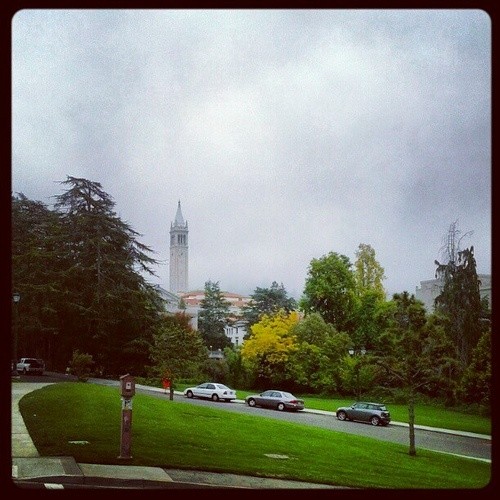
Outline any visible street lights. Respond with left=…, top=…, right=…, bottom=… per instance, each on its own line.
left=348, top=344, right=368, bottom=400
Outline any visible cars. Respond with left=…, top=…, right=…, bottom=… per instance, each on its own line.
left=183, top=382, right=237, bottom=401
left=336, top=401, right=390, bottom=425
left=12, top=357, right=45, bottom=373
left=246, top=390, right=305, bottom=412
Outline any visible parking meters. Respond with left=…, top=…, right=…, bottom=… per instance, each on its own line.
left=119, top=373, right=135, bottom=456
